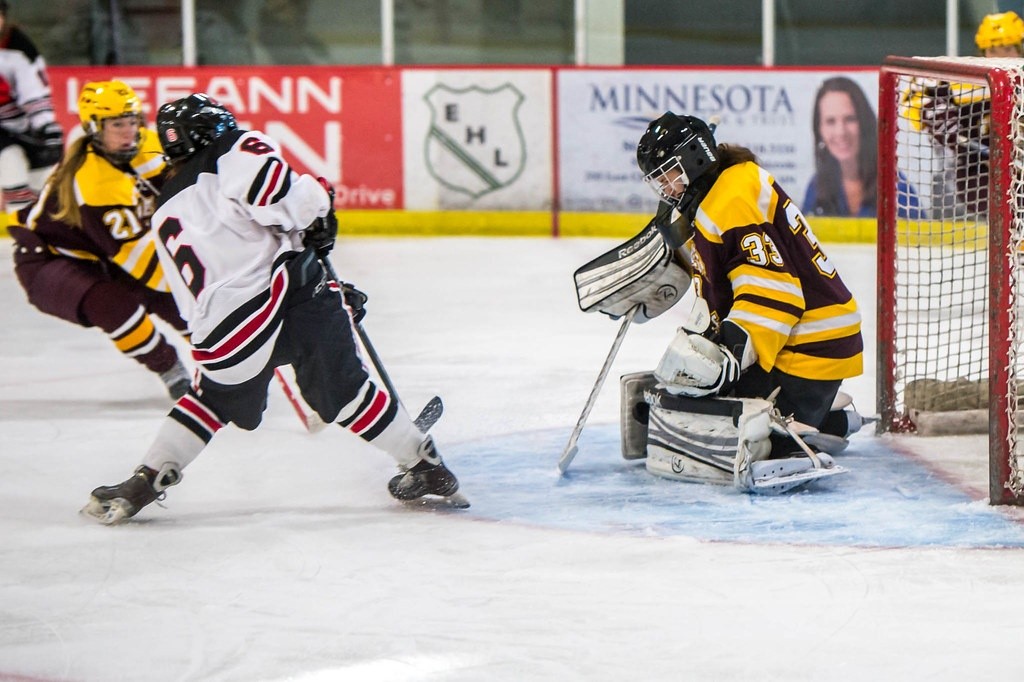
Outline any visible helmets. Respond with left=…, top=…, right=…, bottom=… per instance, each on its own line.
left=155, top=92, right=239, bottom=166
left=77, top=79, right=149, bottom=165
left=974, top=10, right=1024, bottom=50
left=636, top=111, right=720, bottom=213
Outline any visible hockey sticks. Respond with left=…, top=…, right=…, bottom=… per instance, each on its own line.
left=557, top=114, right=721, bottom=472
left=0, top=126, right=40, bottom=146
left=274, top=367, right=327, bottom=433
left=956, top=133, right=991, bottom=157
left=306, top=224, right=443, bottom=435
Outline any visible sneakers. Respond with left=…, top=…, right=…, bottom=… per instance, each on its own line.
left=159, top=359, right=193, bottom=402
left=386, top=434, right=471, bottom=510
left=79, top=461, right=183, bottom=527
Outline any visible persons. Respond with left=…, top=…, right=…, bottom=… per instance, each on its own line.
left=804, top=76, right=924, bottom=221
left=0, top=0, right=65, bottom=212
left=573, top=111, right=864, bottom=495
left=921, top=11, right=1024, bottom=217
left=80, top=93, right=472, bottom=528
left=6, top=82, right=195, bottom=402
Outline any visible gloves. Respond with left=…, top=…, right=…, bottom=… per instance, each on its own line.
left=922, top=101, right=962, bottom=147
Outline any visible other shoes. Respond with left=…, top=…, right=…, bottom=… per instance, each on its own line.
left=819, top=403, right=863, bottom=440
